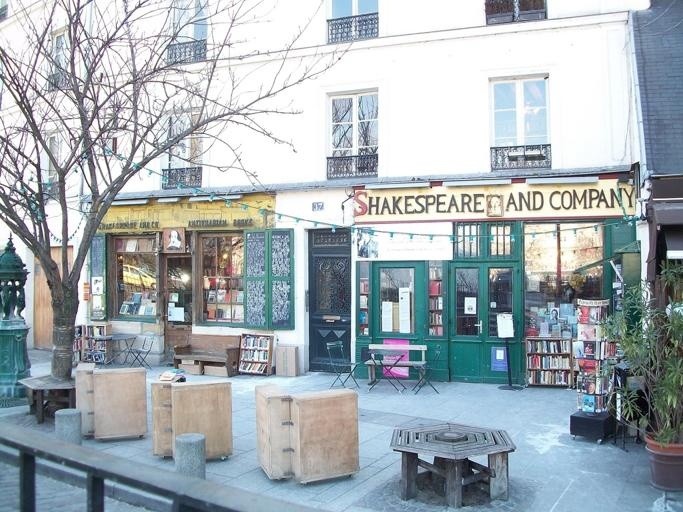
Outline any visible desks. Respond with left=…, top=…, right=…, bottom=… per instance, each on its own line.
left=365, top=349, right=408, bottom=397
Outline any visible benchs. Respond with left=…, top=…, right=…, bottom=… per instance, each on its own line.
left=363, top=344, right=428, bottom=386
left=387, top=416, right=517, bottom=509
left=170, top=332, right=241, bottom=378
left=17, top=373, right=74, bottom=424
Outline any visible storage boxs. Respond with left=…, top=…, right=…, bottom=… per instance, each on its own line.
left=274, top=345, right=298, bottom=377
left=176, top=362, right=228, bottom=377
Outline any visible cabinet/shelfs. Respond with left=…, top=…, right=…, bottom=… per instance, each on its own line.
left=524, top=335, right=577, bottom=390
left=236, top=331, right=277, bottom=376
left=200, top=275, right=245, bottom=320
left=70, top=323, right=112, bottom=367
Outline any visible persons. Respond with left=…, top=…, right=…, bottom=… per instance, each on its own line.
left=167, top=231, right=181, bottom=250
left=551, top=309, right=558, bottom=320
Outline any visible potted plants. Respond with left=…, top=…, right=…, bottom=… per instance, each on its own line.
left=604, top=260, right=683, bottom=494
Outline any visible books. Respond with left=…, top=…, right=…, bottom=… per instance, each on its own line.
left=576, top=305, right=615, bottom=413
left=527, top=340, right=569, bottom=385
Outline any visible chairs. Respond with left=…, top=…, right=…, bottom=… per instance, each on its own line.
left=325, top=340, right=362, bottom=390
left=411, top=344, right=442, bottom=396
left=85, top=334, right=153, bottom=370
